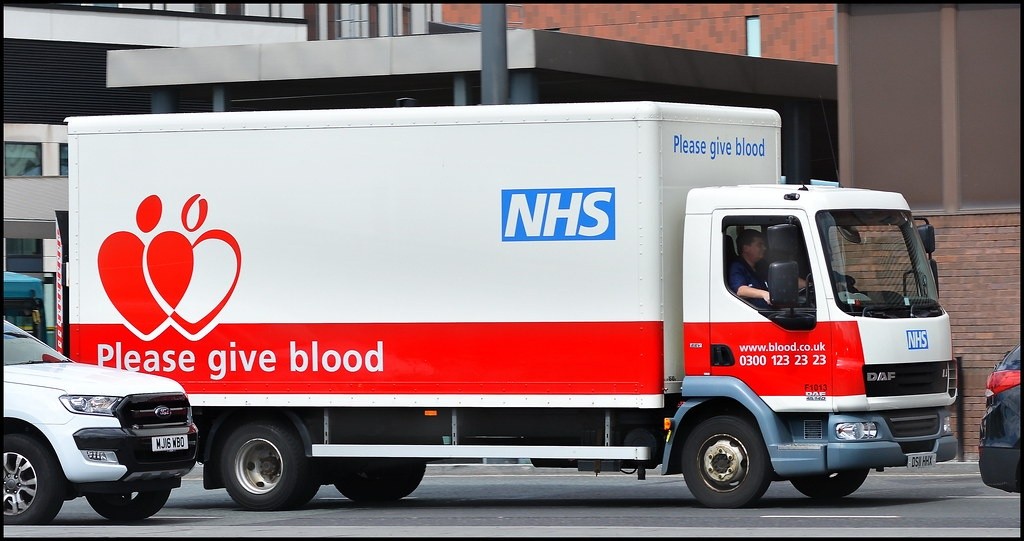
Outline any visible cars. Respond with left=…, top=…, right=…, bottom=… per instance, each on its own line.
left=978, top=342, right=1022, bottom=494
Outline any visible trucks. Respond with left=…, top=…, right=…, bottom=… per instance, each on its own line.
left=59, top=101, right=963, bottom=508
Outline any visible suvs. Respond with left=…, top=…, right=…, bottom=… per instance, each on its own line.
left=0, top=317, right=200, bottom=528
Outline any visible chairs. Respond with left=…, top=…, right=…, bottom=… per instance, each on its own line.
left=724, top=234, right=739, bottom=284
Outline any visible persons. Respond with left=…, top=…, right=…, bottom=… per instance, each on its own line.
left=728, top=229, right=811, bottom=308
